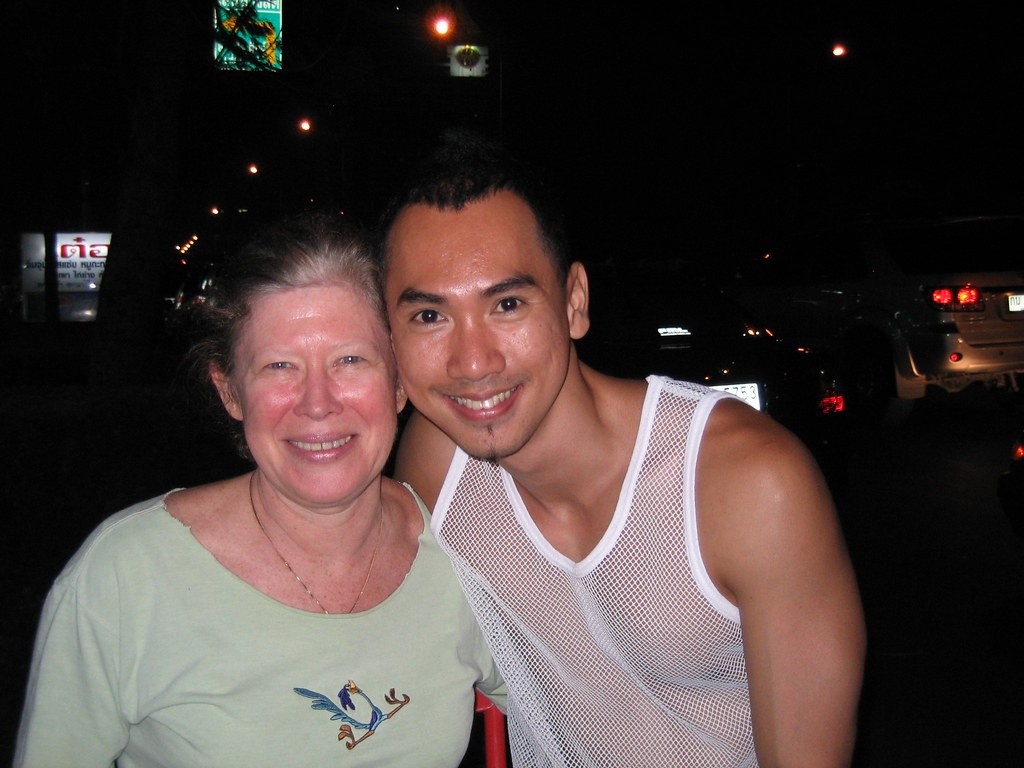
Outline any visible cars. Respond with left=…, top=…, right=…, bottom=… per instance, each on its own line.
left=879, top=214, right=1024, bottom=419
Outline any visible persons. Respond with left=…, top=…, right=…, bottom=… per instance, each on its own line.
left=7, top=214, right=513, bottom=768
left=375, top=129, right=867, bottom=768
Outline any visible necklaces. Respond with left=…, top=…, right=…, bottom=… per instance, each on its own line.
left=246, top=470, right=384, bottom=615
